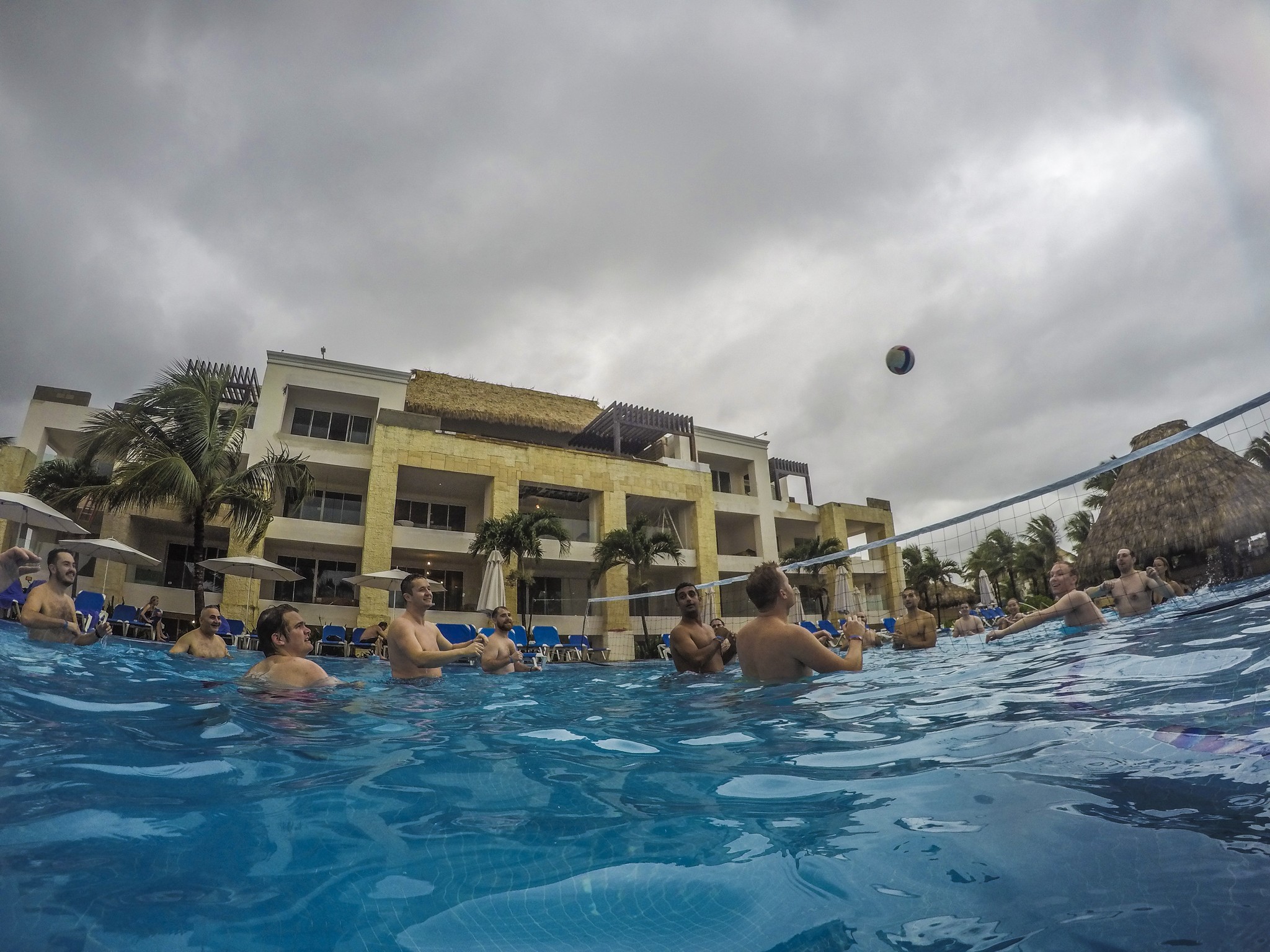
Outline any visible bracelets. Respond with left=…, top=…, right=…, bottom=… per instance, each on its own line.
left=508, top=655, right=514, bottom=663
left=714, top=636, right=724, bottom=641
left=712, top=637, right=722, bottom=644
left=530, top=666, right=535, bottom=672
left=95, top=629, right=103, bottom=639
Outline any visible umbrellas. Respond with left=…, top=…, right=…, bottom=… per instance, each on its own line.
left=58, top=535, right=162, bottom=594
left=0, top=488, right=91, bottom=547
left=340, top=565, right=447, bottom=621
left=196, top=552, right=307, bottom=633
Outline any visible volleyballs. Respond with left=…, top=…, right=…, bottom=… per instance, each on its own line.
left=886, top=345, right=915, bottom=375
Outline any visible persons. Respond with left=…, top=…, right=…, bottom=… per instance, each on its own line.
left=669, top=561, right=936, bottom=683
left=476, top=545, right=506, bottom=629
left=360, top=572, right=489, bottom=678
left=479, top=606, right=543, bottom=675
left=139, top=592, right=230, bottom=657
left=242, top=604, right=343, bottom=687
left=953, top=549, right=1186, bottom=655
left=0, top=546, right=113, bottom=645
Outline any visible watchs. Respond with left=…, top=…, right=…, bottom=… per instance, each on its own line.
left=847, top=635, right=863, bottom=645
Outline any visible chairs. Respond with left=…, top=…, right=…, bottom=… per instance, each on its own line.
left=508, top=625, right=611, bottom=667
left=383, top=645, right=389, bottom=659
left=107, top=604, right=163, bottom=641
left=657, top=633, right=672, bottom=661
left=936, top=627, right=954, bottom=632
left=0, top=578, right=47, bottom=622
left=74, top=590, right=105, bottom=633
left=435, top=623, right=495, bottom=645
left=880, top=617, right=897, bottom=636
left=215, top=614, right=258, bottom=651
left=970, top=607, right=1007, bottom=628
left=315, top=625, right=347, bottom=658
left=798, top=619, right=876, bottom=648
left=347, top=627, right=377, bottom=658
